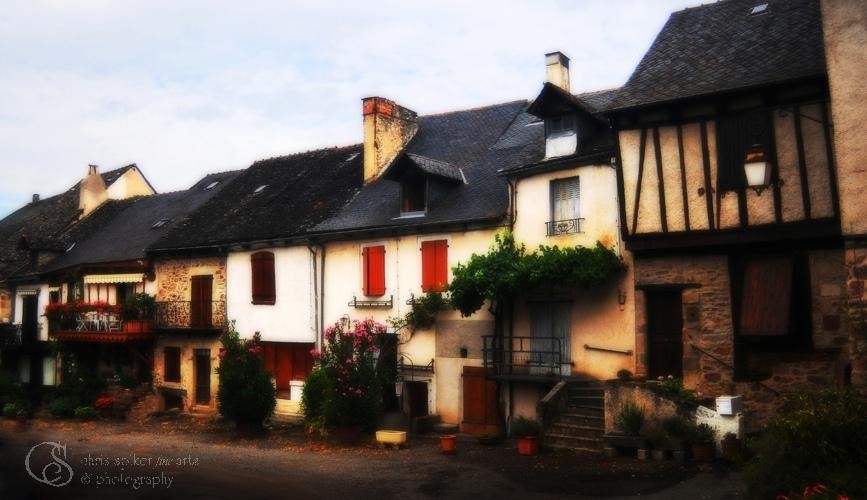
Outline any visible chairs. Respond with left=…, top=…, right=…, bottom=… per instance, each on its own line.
left=75, top=311, right=122, bottom=333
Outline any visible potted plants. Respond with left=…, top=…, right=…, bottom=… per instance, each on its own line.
left=210, top=320, right=279, bottom=438
left=603, top=399, right=741, bottom=464
left=123, top=292, right=157, bottom=334
left=16, top=409, right=28, bottom=423
left=511, top=414, right=543, bottom=455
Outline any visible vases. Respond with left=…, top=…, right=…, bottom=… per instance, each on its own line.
left=440, top=436, right=456, bottom=451
left=57, top=320, right=72, bottom=330
left=335, top=427, right=368, bottom=448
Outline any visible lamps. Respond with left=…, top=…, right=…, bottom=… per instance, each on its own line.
left=743, top=141, right=772, bottom=195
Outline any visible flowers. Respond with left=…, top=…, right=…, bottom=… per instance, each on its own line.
left=311, top=314, right=415, bottom=426
left=43, top=300, right=122, bottom=320
